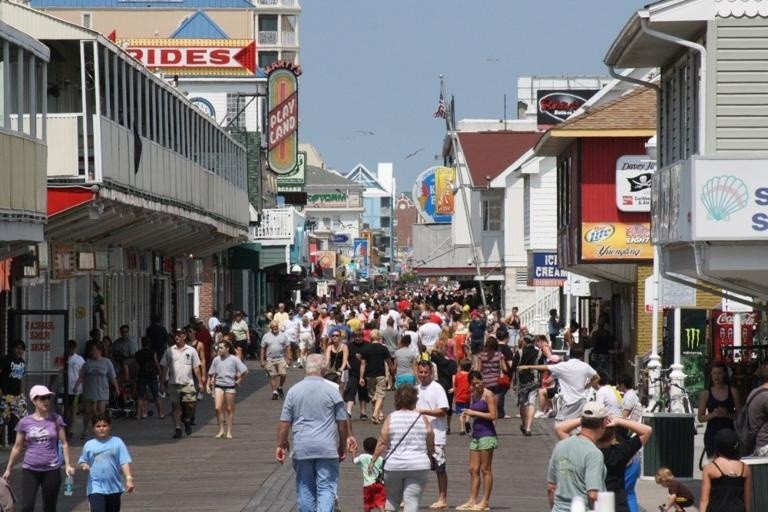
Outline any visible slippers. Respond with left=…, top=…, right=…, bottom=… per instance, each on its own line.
left=472, top=504, right=491, bottom=511
left=456, top=504, right=473, bottom=511
left=429, top=502, right=448, bottom=510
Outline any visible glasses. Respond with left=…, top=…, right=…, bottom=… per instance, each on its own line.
left=35, top=397, right=51, bottom=400
left=332, top=335, right=340, bottom=337
left=121, top=331, right=129, bottom=334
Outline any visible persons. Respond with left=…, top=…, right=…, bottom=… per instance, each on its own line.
left=369, top=385, right=434, bottom=512
left=318, top=330, right=350, bottom=393
left=358, top=328, right=396, bottom=425
left=347, top=437, right=388, bottom=511
left=55, top=340, right=92, bottom=441
left=698, top=429, right=753, bottom=512
left=275, top=353, right=346, bottom=512
left=455, top=370, right=500, bottom=511
left=590, top=313, right=610, bottom=338
left=604, top=425, right=645, bottom=512
left=519, top=328, right=530, bottom=336
left=503, top=306, right=523, bottom=346
left=2, top=383, right=76, bottom=511
left=322, top=371, right=358, bottom=512
left=545, top=400, right=609, bottom=512
left=392, top=334, right=419, bottom=391
left=536, top=354, right=561, bottom=420
left=72, top=343, right=122, bottom=439
left=547, top=308, right=562, bottom=348
left=78, top=413, right=136, bottom=512
left=84, top=329, right=102, bottom=359
left=617, top=373, right=649, bottom=511
left=206, top=339, right=249, bottom=439
left=135, top=336, right=164, bottom=419
left=260, top=321, right=292, bottom=401
left=207, top=309, right=222, bottom=337
left=453, top=358, right=474, bottom=438
left=229, top=310, right=251, bottom=363
left=476, top=337, right=508, bottom=427
left=146, top=315, right=170, bottom=399
left=107, top=367, right=135, bottom=406
left=344, top=328, right=371, bottom=422
left=551, top=410, right=652, bottom=511
left=409, top=360, right=451, bottom=509
left=732, top=371, right=768, bottom=457
left=655, top=466, right=695, bottom=512
left=211, top=332, right=241, bottom=423
left=586, top=368, right=624, bottom=422
left=113, top=325, right=148, bottom=418
left=696, top=361, right=743, bottom=462
left=515, top=345, right=599, bottom=449
left=564, top=320, right=580, bottom=356
left=159, top=328, right=204, bottom=438
left=426, top=343, right=456, bottom=436
left=258, top=281, right=502, bottom=335
left=495, top=329, right=511, bottom=421
left=101, top=336, right=111, bottom=356
left=589, top=319, right=613, bottom=352
left=180, top=323, right=206, bottom=425
left=511, top=334, right=542, bottom=437
left=529, top=334, right=551, bottom=414
left=0, top=337, right=32, bottom=447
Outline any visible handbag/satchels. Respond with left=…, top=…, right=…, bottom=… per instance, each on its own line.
left=499, top=354, right=510, bottom=387
left=52, top=414, right=65, bottom=467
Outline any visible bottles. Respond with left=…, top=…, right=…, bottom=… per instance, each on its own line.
left=459, top=415, right=466, bottom=438
left=64, top=471, right=74, bottom=498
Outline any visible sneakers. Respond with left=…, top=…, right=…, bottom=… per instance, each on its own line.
left=360, top=413, right=368, bottom=420
left=372, top=416, right=382, bottom=425
left=347, top=411, right=351, bottom=418
left=379, top=416, right=385, bottom=421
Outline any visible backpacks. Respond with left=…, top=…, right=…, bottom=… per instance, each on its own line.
left=733, top=386, right=765, bottom=459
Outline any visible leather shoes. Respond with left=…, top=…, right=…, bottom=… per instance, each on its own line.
left=272, top=394, right=278, bottom=400
left=278, top=387, right=284, bottom=397
left=173, top=428, right=183, bottom=438
left=185, top=426, right=192, bottom=435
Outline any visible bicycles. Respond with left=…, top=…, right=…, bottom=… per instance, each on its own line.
left=646, top=368, right=685, bottom=413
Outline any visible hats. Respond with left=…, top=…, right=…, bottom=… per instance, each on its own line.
left=30, top=385, right=55, bottom=400
left=288, top=310, right=295, bottom=314
left=174, top=328, right=187, bottom=335
left=581, top=403, right=609, bottom=418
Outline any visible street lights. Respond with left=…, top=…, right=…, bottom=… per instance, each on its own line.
left=646, top=134, right=660, bottom=409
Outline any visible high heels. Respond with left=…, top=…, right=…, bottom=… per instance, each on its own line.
left=216, top=432, right=226, bottom=438
left=226, top=433, right=232, bottom=439
